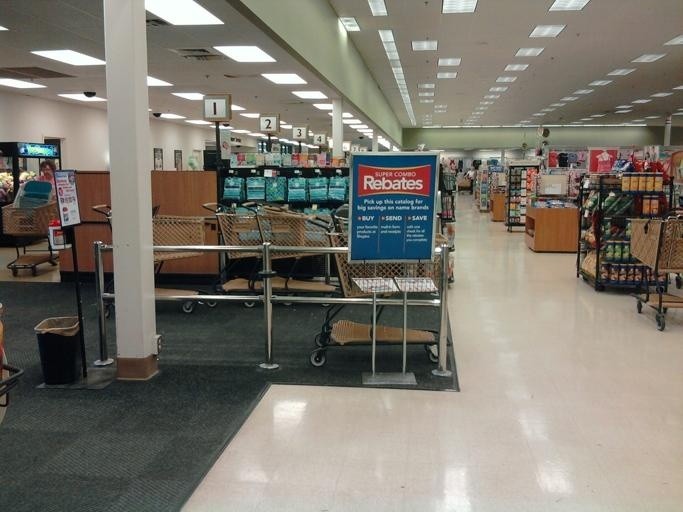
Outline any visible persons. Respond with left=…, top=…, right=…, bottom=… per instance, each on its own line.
left=35, top=159, right=57, bottom=201
left=455, top=165, right=477, bottom=195
left=645, top=146, right=657, bottom=162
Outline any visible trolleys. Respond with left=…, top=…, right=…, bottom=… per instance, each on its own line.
left=458, top=176, right=473, bottom=195
left=1, top=194, right=59, bottom=277
left=624, top=215, right=683, bottom=331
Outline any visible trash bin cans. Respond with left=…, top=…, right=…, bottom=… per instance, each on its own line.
left=34, top=316, right=83, bottom=385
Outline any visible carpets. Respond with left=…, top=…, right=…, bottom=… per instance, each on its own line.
left=0, top=281, right=461, bottom=512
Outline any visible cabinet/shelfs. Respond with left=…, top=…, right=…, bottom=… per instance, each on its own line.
left=489, top=193, right=505, bottom=222
left=525, top=201, right=580, bottom=253
left=577, top=173, right=674, bottom=292
left=506, top=166, right=540, bottom=233
left=440, top=164, right=455, bottom=287
